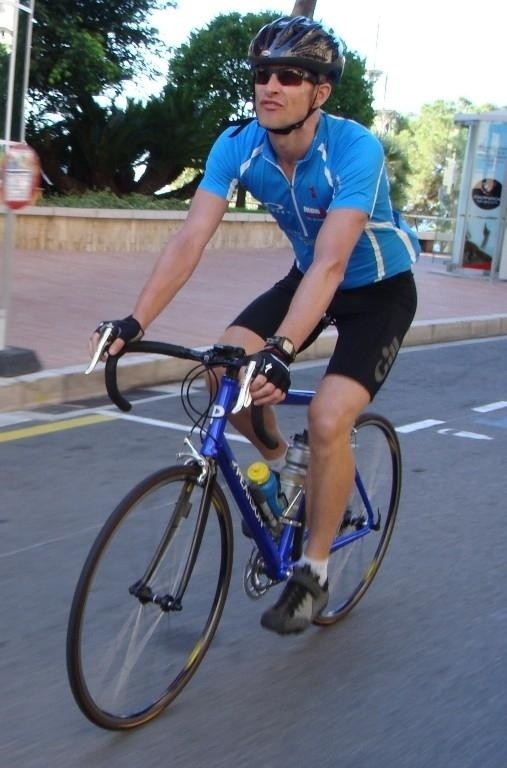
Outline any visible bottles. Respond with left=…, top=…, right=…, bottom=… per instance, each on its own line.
left=247, top=461, right=283, bottom=520
left=279, top=428, right=309, bottom=508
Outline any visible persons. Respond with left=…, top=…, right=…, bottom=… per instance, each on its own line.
left=80, top=13, right=422, bottom=637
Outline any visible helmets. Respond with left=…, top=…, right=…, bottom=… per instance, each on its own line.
left=247, top=15, right=346, bottom=82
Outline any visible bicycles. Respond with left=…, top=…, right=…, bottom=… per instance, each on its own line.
left=66, top=324, right=402, bottom=732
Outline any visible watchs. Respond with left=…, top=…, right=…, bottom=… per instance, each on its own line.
left=264, top=335, right=297, bottom=364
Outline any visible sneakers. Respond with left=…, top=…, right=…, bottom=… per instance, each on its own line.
left=261, top=562, right=329, bottom=636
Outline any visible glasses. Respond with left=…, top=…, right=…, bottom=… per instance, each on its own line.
left=253, top=68, right=316, bottom=86
